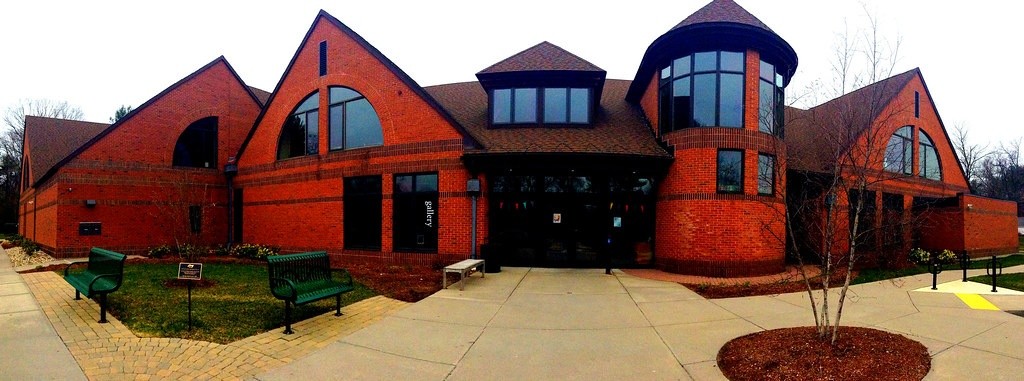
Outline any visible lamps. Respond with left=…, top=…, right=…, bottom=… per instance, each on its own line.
left=86, top=200, right=96, bottom=206
left=968, top=203, right=973, bottom=210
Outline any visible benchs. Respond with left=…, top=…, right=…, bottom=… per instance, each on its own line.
left=267, top=252, right=355, bottom=337
left=64, top=247, right=127, bottom=324
left=442, top=259, right=485, bottom=291
